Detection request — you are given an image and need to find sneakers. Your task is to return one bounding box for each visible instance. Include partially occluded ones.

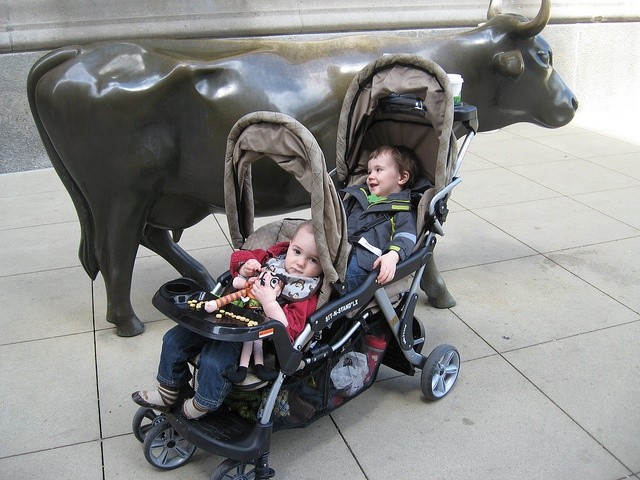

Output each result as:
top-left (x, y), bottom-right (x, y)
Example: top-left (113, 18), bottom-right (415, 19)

top-left (182, 397), bottom-right (208, 420)
top-left (132, 389), bottom-right (174, 414)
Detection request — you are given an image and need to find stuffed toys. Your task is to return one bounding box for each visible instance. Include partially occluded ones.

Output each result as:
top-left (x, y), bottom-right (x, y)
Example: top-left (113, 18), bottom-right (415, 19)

top-left (204, 263), bottom-right (286, 383)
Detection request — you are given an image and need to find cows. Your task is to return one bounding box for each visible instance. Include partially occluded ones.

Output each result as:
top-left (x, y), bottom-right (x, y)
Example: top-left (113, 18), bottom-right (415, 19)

top-left (25, 0), bottom-right (579, 336)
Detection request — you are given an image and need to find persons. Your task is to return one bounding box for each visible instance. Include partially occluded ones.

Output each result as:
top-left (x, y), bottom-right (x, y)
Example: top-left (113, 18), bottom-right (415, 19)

top-left (343, 144), bottom-right (421, 292)
top-left (131, 220), bottom-right (324, 420)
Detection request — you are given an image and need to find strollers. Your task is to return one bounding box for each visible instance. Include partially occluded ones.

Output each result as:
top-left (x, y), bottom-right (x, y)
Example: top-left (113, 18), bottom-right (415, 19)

top-left (132, 53), bottom-right (480, 479)
